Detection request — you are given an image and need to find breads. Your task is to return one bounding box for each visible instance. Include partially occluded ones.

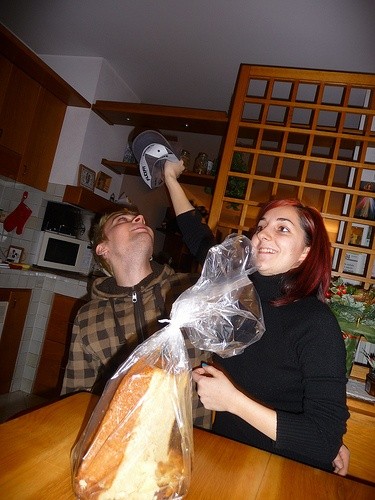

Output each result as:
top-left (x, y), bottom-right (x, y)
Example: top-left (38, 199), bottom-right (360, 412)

top-left (73, 352), bottom-right (186, 500)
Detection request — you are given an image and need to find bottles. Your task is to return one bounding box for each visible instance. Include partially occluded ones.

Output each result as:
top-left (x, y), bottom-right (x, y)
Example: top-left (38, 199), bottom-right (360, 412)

top-left (365, 368), bottom-right (375, 396)
top-left (205, 159), bottom-right (220, 175)
top-left (193, 152), bottom-right (207, 175)
top-left (180, 151), bottom-right (191, 175)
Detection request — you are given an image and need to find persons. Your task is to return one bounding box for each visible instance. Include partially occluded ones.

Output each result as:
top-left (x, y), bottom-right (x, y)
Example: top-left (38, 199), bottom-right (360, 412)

top-left (61, 209), bottom-right (350, 476)
top-left (164, 160), bottom-right (350, 472)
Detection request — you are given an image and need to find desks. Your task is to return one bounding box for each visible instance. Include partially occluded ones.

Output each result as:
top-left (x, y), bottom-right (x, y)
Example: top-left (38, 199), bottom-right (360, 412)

top-left (0, 391), bottom-right (375, 500)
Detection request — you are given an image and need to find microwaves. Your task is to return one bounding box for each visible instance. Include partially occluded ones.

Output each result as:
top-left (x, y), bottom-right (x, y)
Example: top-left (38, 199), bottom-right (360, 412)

top-left (36, 230), bottom-right (96, 276)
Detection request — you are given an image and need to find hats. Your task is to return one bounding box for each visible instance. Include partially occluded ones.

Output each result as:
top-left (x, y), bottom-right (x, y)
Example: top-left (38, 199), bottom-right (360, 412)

top-left (131, 129), bottom-right (179, 190)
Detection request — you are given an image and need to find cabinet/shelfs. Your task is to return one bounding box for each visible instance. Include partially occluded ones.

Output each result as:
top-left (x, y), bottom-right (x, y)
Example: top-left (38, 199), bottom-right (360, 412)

top-left (0, 25), bottom-right (92, 191)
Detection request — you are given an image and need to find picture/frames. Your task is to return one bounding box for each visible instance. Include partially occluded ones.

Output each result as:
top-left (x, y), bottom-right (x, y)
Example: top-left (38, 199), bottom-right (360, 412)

top-left (78, 164), bottom-right (96, 192)
top-left (4, 245), bottom-right (25, 264)
